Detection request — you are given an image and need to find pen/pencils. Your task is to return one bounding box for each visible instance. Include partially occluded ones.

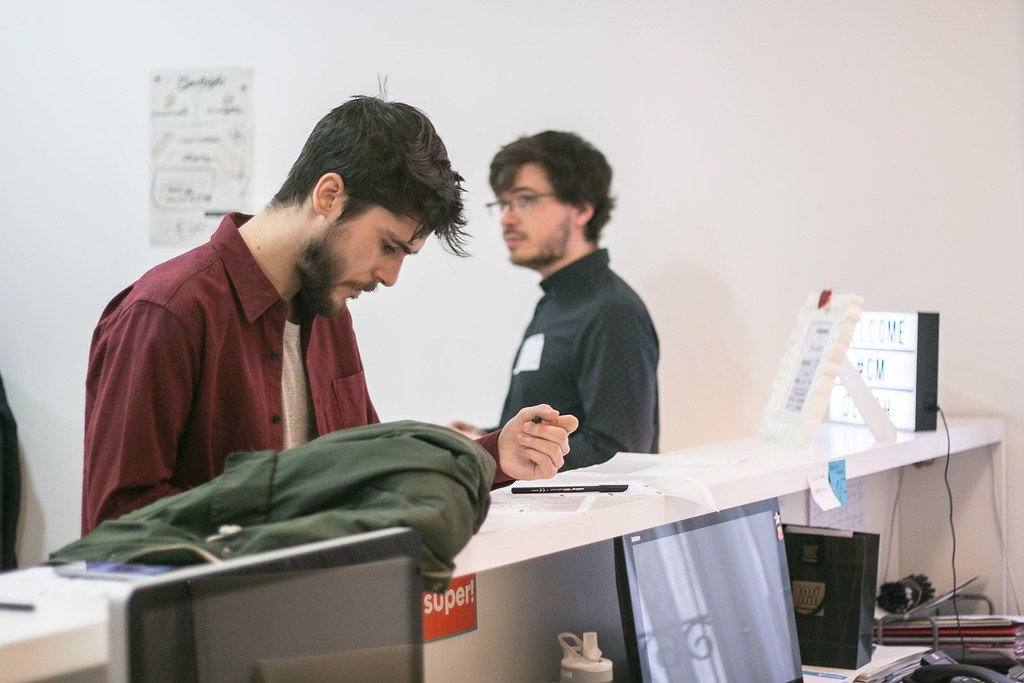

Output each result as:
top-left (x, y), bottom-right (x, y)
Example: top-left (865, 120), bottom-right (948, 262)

top-left (531, 416), bottom-right (542, 424)
top-left (0, 603), bottom-right (33, 612)
top-left (511, 485), bottom-right (629, 494)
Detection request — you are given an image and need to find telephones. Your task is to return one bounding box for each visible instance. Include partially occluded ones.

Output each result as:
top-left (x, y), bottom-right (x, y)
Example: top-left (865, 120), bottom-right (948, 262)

top-left (902, 650), bottom-right (1024, 683)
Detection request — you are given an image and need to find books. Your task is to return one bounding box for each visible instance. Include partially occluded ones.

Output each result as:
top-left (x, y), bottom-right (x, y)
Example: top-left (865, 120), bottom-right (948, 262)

top-left (802, 614), bottom-right (1024, 683)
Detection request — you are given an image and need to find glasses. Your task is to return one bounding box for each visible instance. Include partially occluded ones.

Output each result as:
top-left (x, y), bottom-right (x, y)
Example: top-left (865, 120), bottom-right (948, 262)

top-left (486, 192), bottom-right (557, 216)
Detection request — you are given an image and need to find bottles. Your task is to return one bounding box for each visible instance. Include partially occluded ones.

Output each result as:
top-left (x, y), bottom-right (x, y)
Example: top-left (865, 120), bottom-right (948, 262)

top-left (557, 629), bottom-right (613, 683)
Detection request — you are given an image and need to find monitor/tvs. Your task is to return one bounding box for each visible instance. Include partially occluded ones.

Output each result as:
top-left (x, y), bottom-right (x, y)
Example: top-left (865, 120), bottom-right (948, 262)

top-left (614, 496), bottom-right (806, 683)
top-left (105, 525), bottom-right (430, 683)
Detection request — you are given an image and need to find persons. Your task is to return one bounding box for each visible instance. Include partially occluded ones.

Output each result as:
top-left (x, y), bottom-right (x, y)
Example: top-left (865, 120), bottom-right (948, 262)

top-left (446, 130), bottom-right (660, 474)
top-left (81, 74), bottom-right (578, 537)
top-left (0, 375), bottom-right (20, 574)
